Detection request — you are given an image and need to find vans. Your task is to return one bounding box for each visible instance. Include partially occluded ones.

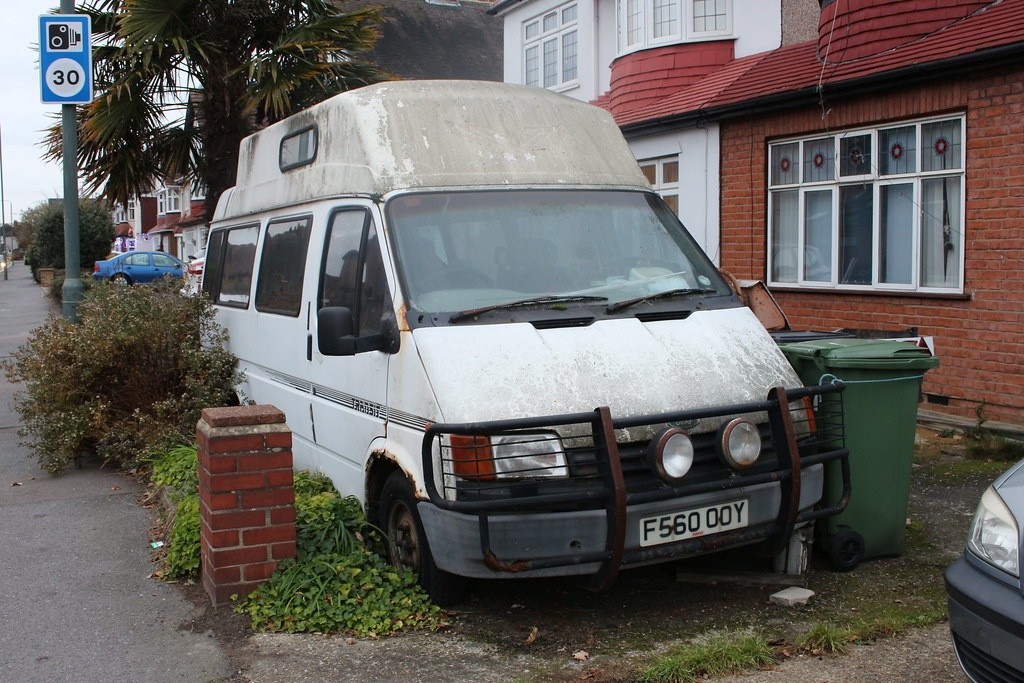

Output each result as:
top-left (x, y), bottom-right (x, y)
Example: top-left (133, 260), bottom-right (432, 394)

top-left (197, 80), bottom-right (824, 604)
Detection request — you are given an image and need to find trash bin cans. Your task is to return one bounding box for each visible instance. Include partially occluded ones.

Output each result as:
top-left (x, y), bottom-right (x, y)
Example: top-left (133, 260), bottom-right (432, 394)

top-left (774, 338), bottom-right (939, 573)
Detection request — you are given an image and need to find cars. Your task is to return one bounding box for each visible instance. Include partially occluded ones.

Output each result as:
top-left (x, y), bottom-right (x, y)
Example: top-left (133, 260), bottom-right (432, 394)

top-left (93, 251), bottom-right (190, 287)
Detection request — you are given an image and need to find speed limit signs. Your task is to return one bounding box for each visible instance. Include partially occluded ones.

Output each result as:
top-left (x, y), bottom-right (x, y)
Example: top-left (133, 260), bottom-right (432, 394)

top-left (39, 14), bottom-right (94, 105)
